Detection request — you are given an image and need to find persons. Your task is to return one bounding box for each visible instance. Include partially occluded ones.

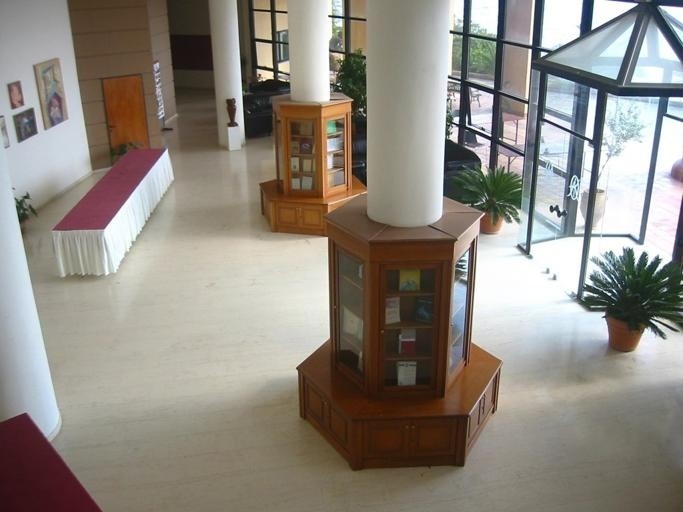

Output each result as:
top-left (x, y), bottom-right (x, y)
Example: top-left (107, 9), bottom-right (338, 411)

top-left (9, 83), bottom-right (22, 109)
top-left (48, 95), bottom-right (63, 124)
top-left (14, 114), bottom-right (33, 139)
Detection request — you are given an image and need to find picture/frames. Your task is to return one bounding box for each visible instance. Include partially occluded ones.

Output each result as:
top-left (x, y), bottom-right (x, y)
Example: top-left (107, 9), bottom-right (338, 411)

top-left (5, 57), bottom-right (70, 143)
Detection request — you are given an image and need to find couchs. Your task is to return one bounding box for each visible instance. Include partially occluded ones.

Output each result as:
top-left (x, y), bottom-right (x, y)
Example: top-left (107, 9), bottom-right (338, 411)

top-left (242, 78), bottom-right (291, 136)
top-left (353, 136), bottom-right (481, 206)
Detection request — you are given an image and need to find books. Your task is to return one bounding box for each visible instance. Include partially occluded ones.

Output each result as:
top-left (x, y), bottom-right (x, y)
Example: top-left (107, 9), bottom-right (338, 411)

top-left (286, 120), bottom-right (340, 192)
top-left (398, 269), bottom-right (421, 291)
top-left (342, 307), bottom-right (363, 346)
top-left (355, 351), bottom-right (364, 371)
top-left (396, 360), bottom-right (417, 386)
top-left (384, 297), bottom-right (400, 324)
top-left (398, 329), bottom-right (415, 358)
top-left (413, 297), bottom-right (433, 325)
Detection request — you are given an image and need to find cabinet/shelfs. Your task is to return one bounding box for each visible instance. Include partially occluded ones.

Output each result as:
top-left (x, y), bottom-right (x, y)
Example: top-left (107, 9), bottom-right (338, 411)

top-left (324, 224), bottom-right (476, 401)
top-left (267, 95), bottom-right (351, 199)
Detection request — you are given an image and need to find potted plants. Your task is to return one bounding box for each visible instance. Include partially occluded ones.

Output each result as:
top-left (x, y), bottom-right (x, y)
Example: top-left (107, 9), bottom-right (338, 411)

top-left (579, 97), bottom-right (646, 232)
top-left (450, 162), bottom-right (524, 234)
top-left (570, 245), bottom-right (683, 353)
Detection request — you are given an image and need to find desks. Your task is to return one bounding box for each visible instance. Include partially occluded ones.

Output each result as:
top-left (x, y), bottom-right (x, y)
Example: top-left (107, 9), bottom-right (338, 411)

top-left (51, 146), bottom-right (171, 280)
